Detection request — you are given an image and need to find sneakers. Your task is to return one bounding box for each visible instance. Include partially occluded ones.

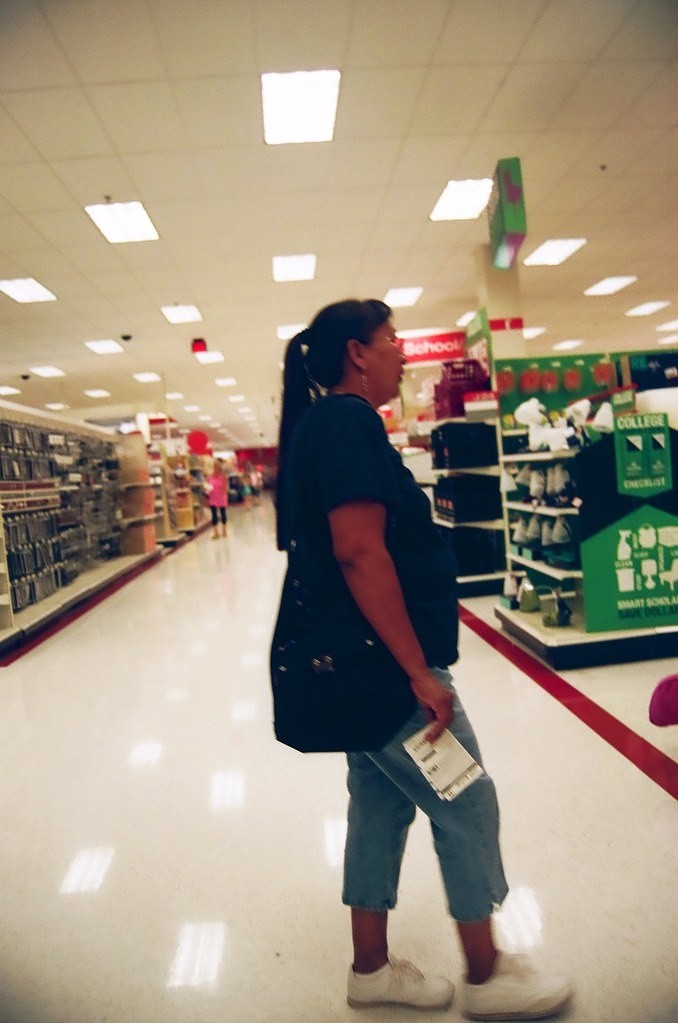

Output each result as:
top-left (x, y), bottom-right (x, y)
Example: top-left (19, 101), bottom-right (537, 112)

top-left (346, 953), bottom-right (455, 1010)
top-left (460, 949), bottom-right (572, 1021)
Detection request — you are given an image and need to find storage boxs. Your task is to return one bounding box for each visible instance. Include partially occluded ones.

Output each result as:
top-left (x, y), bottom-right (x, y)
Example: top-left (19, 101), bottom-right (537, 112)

top-left (116, 466), bottom-right (157, 554)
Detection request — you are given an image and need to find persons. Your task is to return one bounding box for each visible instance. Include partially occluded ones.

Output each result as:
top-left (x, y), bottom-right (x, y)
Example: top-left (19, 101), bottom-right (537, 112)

top-left (213, 298), bottom-right (576, 1022)
top-left (202, 457), bottom-right (263, 541)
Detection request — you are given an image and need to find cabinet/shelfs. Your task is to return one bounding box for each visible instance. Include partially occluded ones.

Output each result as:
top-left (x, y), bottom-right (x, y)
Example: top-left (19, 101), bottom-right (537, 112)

top-left (148, 443), bottom-right (224, 544)
top-left (375, 304), bottom-right (678, 671)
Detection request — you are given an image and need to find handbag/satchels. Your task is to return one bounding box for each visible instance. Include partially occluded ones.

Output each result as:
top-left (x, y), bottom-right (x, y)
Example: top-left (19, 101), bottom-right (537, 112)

top-left (271, 529), bottom-right (418, 753)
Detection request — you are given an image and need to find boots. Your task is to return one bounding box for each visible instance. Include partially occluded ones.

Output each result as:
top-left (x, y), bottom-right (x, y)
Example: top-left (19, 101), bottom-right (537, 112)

top-left (221, 524), bottom-right (227, 537)
top-left (211, 525), bottom-right (219, 540)
